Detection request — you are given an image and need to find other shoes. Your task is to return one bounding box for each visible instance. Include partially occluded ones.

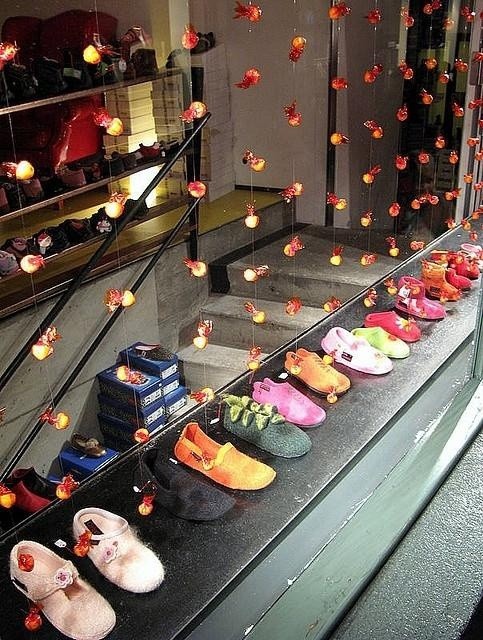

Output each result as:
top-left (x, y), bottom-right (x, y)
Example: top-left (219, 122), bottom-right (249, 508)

top-left (222, 392), bottom-right (312, 458)
top-left (365, 311), bottom-right (422, 343)
top-left (321, 328), bottom-right (394, 375)
top-left (284, 348), bottom-right (350, 395)
top-left (353, 325), bottom-right (411, 360)
top-left (252, 378), bottom-right (326, 428)
top-left (173, 420), bottom-right (278, 491)
top-left (432, 244), bottom-right (482, 290)
top-left (134, 447), bottom-right (236, 520)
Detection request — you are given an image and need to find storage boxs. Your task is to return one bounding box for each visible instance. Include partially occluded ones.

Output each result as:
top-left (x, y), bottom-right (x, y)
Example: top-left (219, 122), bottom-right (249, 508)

top-left (48, 338), bottom-right (188, 487)
top-left (103, 39), bottom-right (239, 203)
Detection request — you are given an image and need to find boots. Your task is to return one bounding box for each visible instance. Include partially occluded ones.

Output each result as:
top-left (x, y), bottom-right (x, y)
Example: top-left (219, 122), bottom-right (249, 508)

top-left (420, 261), bottom-right (461, 302)
top-left (2, 467), bottom-right (58, 512)
top-left (397, 276), bottom-right (448, 320)
top-left (0, 49), bottom-right (158, 105)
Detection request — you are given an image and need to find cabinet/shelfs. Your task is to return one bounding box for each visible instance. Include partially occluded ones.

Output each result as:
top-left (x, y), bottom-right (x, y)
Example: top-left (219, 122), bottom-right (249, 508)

top-left (0, 63), bottom-right (205, 320)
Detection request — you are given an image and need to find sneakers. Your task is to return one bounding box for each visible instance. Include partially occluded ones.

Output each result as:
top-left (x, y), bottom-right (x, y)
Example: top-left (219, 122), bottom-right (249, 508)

top-left (0, 140), bottom-right (179, 216)
top-left (134, 343), bottom-right (176, 361)
top-left (71, 432), bottom-right (106, 457)
top-left (0, 198), bottom-right (149, 276)
top-left (10, 506), bottom-right (165, 640)
